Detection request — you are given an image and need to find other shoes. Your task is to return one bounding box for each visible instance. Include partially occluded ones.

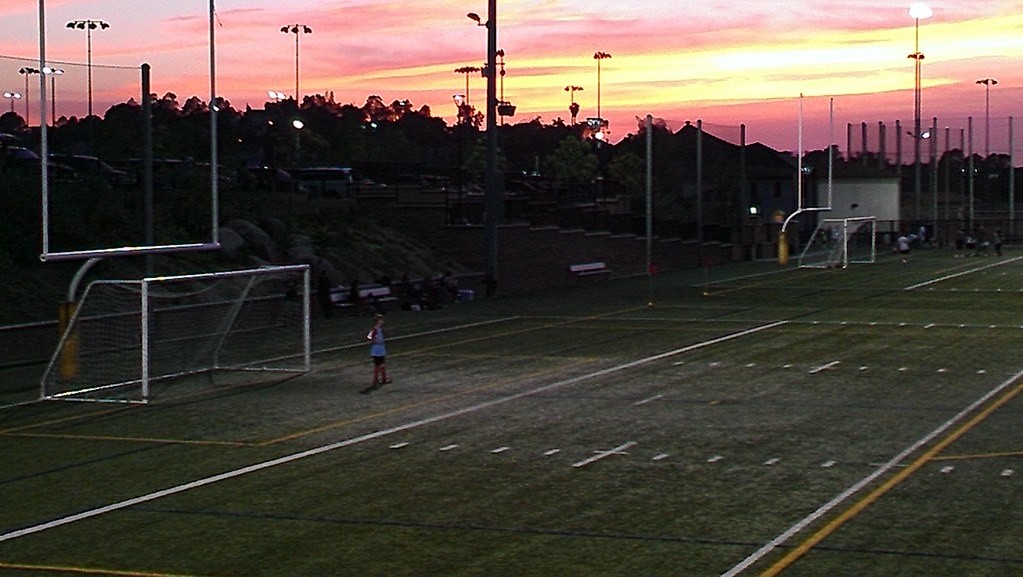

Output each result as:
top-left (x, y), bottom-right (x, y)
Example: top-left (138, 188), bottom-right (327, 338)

top-left (381, 378), bottom-right (392, 384)
top-left (374, 381), bottom-right (384, 387)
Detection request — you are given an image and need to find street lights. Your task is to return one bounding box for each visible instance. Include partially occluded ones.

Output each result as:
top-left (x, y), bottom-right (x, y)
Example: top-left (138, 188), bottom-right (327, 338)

top-left (564, 85), bottom-right (585, 125)
top-left (975, 78), bottom-right (998, 158)
top-left (18, 65), bottom-right (64, 127)
top-left (594, 50), bottom-right (614, 118)
top-left (907, 3), bottom-right (934, 222)
top-left (64, 16), bottom-right (110, 115)
top-left (279, 22), bottom-right (313, 165)
top-left (467, 12), bottom-right (501, 302)
top-left (3, 92), bottom-right (22, 113)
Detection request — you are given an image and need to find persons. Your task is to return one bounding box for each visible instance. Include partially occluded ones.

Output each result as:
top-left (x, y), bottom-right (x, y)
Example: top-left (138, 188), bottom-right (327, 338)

top-left (400, 274), bottom-right (426, 310)
top-left (319, 270), bottom-right (339, 319)
top-left (897, 233), bottom-right (910, 263)
top-left (954, 228), bottom-right (965, 258)
top-left (993, 231), bottom-right (1002, 256)
top-left (883, 231), bottom-right (892, 249)
top-left (365, 314), bottom-right (392, 386)
top-left (349, 280), bottom-right (388, 317)
top-left (965, 230), bottom-right (978, 256)
top-left (440, 271), bottom-right (459, 303)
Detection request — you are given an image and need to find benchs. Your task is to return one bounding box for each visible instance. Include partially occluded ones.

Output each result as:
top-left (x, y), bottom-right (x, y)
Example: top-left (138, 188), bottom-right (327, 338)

top-left (331, 287), bottom-right (400, 317)
top-left (569, 262), bottom-right (613, 281)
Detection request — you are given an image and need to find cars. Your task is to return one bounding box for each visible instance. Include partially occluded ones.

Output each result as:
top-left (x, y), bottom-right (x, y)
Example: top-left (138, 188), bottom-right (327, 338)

top-left (1, 130), bottom-right (605, 213)
